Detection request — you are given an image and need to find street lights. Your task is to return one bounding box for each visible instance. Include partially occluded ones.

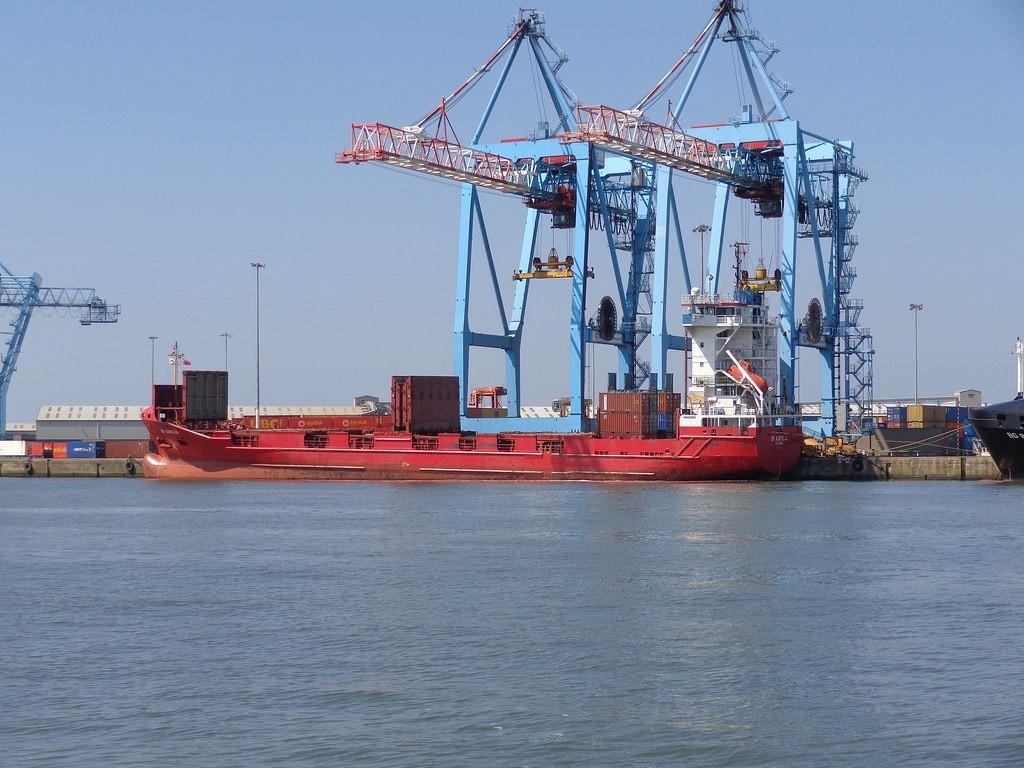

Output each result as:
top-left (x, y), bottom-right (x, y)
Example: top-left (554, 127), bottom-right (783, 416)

top-left (251, 260), bottom-right (266, 429)
top-left (150, 336), bottom-right (159, 385)
top-left (694, 225), bottom-right (710, 296)
top-left (909, 303), bottom-right (923, 405)
top-left (221, 333), bottom-right (233, 371)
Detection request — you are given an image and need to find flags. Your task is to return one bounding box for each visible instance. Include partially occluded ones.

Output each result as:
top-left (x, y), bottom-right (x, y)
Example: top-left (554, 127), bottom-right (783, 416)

top-left (183, 358), bottom-right (191, 365)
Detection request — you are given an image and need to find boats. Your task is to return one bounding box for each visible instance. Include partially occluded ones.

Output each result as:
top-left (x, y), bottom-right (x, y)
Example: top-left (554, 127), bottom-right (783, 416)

top-left (140, 341), bottom-right (804, 484)
top-left (967, 338), bottom-right (1024, 482)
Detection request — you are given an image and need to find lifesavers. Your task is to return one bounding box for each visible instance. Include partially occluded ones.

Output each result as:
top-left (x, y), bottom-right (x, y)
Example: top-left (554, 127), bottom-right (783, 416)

top-left (851, 460), bottom-right (864, 472)
